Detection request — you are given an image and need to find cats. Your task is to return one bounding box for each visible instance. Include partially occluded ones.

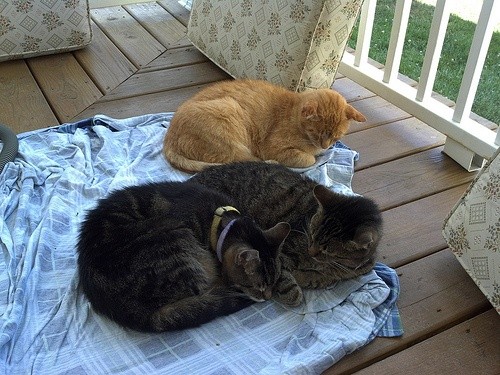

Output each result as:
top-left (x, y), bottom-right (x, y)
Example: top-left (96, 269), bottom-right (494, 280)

top-left (161, 80), bottom-right (367, 172)
top-left (74, 159), bottom-right (384, 334)
